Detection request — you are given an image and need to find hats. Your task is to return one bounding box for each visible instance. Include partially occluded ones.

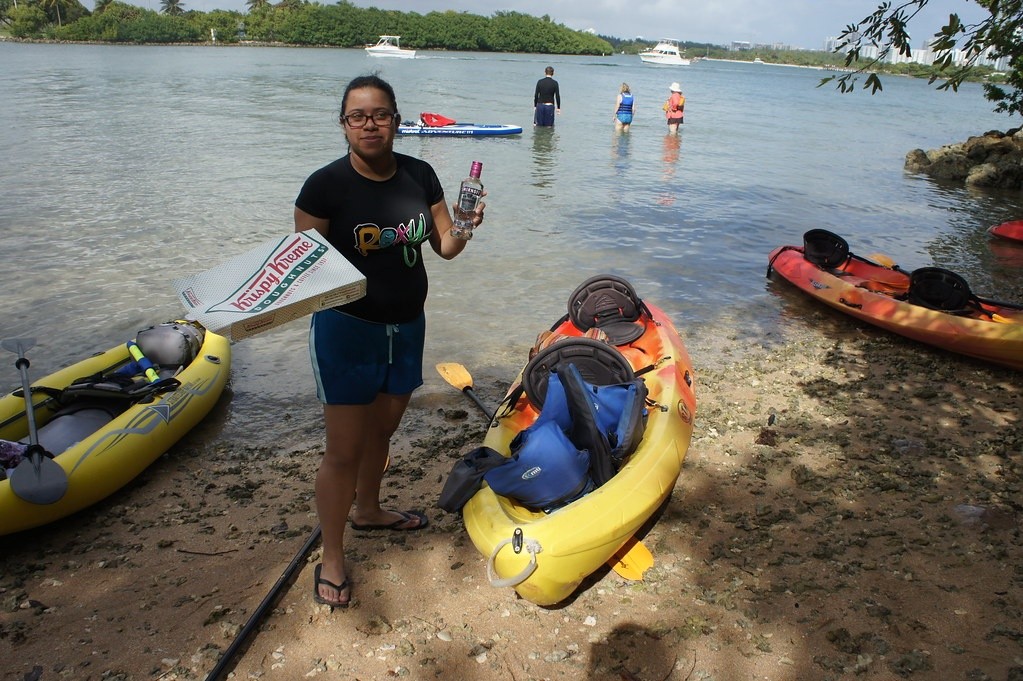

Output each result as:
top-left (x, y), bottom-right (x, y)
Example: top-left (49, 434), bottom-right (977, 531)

top-left (669, 83), bottom-right (682, 93)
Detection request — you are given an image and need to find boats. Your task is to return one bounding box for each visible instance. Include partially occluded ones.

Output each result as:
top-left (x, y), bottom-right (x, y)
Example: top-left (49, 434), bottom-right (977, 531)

top-left (986, 219), bottom-right (1023, 244)
top-left (395, 111), bottom-right (524, 140)
top-left (765, 227), bottom-right (1023, 374)
top-left (459, 272), bottom-right (698, 610)
top-left (0, 321), bottom-right (230, 539)
top-left (364, 33), bottom-right (417, 59)
top-left (639, 37), bottom-right (691, 67)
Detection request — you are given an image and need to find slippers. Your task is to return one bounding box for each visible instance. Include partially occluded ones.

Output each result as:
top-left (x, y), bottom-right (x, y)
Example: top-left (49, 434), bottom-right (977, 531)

top-left (352, 509), bottom-right (428, 530)
top-left (313, 563), bottom-right (351, 606)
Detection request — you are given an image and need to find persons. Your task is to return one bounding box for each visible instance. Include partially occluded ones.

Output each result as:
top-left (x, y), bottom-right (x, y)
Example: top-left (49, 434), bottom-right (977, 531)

top-left (665, 82), bottom-right (686, 134)
top-left (612, 81), bottom-right (636, 130)
top-left (534, 65), bottom-right (561, 125)
top-left (295, 74), bottom-right (490, 605)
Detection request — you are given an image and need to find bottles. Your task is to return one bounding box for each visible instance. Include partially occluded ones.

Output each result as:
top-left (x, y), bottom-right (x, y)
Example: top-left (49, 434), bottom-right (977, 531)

top-left (450, 160), bottom-right (484, 240)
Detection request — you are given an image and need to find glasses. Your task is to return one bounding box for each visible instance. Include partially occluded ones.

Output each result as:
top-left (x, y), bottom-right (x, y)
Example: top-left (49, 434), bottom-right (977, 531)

top-left (341, 112), bottom-right (394, 128)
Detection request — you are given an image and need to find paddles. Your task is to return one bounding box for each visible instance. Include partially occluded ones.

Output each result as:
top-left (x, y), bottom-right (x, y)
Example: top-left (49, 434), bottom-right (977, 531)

top-left (867, 252), bottom-right (1014, 326)
top-left (436, 357), bottom-right (656, 585)
top-left (201, 451), bottom-right (395, 681)
